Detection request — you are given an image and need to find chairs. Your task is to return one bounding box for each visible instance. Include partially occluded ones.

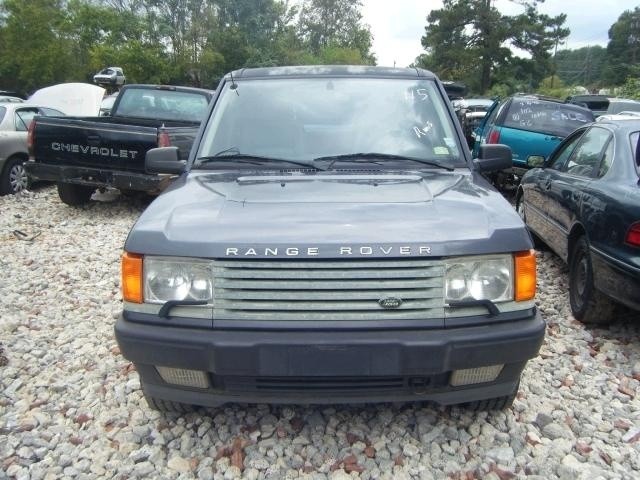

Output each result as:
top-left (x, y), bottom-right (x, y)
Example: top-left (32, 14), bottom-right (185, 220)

top-left (241, 105), bottom-right (308, 158)
